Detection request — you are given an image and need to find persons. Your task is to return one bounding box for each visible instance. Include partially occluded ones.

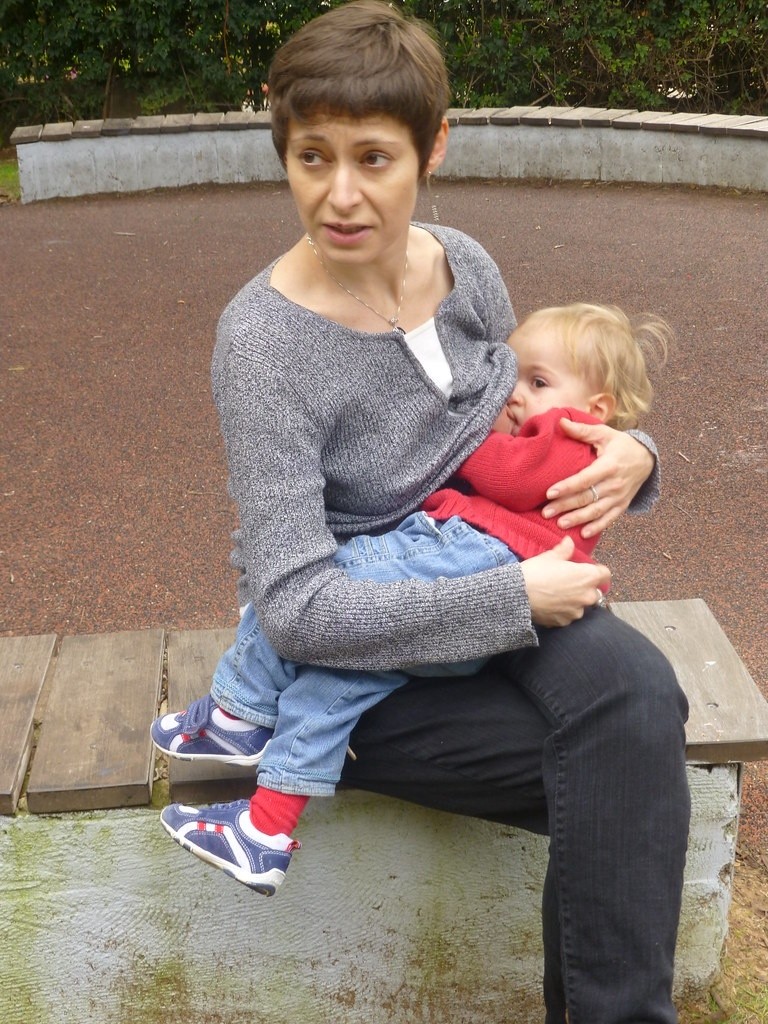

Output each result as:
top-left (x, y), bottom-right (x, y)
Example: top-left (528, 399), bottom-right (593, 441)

top-left (149, 298), bottom-right (671, 894)
top-left (207, 0), bottom-right (691, 1024)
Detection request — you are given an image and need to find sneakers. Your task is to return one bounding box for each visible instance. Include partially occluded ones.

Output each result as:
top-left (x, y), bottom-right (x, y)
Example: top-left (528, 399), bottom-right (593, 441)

top-left (150, 694), bottom-right (272, 764)
top-left (160, 799), bottom-right (301, 899)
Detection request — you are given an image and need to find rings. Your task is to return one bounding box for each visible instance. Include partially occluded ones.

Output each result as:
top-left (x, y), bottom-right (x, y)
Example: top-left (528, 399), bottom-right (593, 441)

top-left (590, 486), bottom-right (600, 501)
top-left (597, 588), bottom-right (605, 608)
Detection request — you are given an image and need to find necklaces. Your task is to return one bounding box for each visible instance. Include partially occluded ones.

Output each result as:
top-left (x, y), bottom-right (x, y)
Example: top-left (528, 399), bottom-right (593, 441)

top-left (307, 229), bottom-right (414, 334)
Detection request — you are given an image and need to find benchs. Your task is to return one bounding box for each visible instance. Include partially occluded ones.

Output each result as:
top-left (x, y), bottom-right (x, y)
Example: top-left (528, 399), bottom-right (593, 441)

top-left (1, 598), bottom-right (767, 1024)
top-left (11, 107), bottom-right (768, 203)
